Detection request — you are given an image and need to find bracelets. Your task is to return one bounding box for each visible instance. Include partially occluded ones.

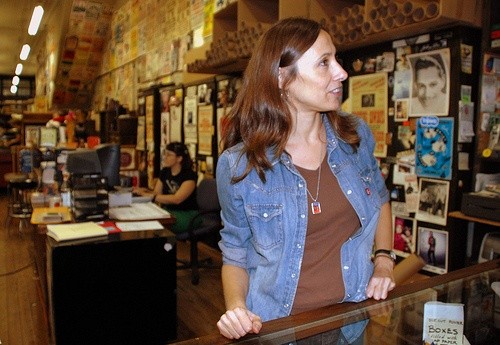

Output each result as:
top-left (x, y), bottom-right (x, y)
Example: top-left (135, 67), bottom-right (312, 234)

top-left (375, 249), bottom-right (396, 260)
top-left (152, 194), bottom-right (157, 202)
top-left (371, 255), bottom-right (393, 263)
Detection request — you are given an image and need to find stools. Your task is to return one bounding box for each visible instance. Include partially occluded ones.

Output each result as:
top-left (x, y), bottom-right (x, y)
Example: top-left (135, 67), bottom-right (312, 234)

top-left (5, 171), bottom-right (35, 219)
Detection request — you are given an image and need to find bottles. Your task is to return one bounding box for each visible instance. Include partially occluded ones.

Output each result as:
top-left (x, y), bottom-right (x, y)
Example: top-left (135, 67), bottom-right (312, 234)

top-left (61, 175), bottom-right (72, 212)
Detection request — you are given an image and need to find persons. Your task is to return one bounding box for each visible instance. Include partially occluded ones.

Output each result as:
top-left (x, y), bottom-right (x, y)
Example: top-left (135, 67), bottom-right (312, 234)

top-left (133, 141), bottom-right (203, 233)
top-left (427, 231), bottom-right (437, 266)
top-left (394, 218), bottom-right (412, 253)
top-left (215, 16), bottom-right (396, 345)
top-left (395, 49), bottom-right (448, 115)
top-left (419, 185), bottom-right (446, 217)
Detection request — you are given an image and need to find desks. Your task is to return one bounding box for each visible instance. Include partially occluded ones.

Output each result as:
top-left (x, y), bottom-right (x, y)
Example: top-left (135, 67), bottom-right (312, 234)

top-left (32, 219), bottom-right (177, 344)
top-left (448, 210), bottom-right (500, 257)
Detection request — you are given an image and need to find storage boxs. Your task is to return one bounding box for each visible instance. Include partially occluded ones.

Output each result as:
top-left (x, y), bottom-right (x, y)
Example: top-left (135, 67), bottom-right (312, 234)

top-left (464, 190), bottom-right (500, 222)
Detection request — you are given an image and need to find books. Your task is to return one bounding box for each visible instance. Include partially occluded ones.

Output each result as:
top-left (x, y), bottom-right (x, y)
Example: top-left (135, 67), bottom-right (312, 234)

top-left (45, 220), bottom-right (109, 248)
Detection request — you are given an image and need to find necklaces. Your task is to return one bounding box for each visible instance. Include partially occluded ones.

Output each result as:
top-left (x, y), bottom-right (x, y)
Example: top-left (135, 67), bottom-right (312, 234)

top-left (287, 124), bottom-right (325, 215)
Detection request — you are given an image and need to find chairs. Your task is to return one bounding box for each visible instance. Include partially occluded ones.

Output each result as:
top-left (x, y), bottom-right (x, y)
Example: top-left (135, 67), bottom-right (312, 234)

top-left (176, 178), bottom-right (223, 286)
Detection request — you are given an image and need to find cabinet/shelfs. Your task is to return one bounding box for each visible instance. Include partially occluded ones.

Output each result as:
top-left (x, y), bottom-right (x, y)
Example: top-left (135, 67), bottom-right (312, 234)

top-left (186, 0), bottom-right (483, 75)
top-left (98, 109), bottom-right (137, 145)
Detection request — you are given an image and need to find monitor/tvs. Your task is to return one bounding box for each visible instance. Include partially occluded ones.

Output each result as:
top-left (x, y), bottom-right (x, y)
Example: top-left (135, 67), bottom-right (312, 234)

top-left (66, 143), bottom-right (121, 192)
